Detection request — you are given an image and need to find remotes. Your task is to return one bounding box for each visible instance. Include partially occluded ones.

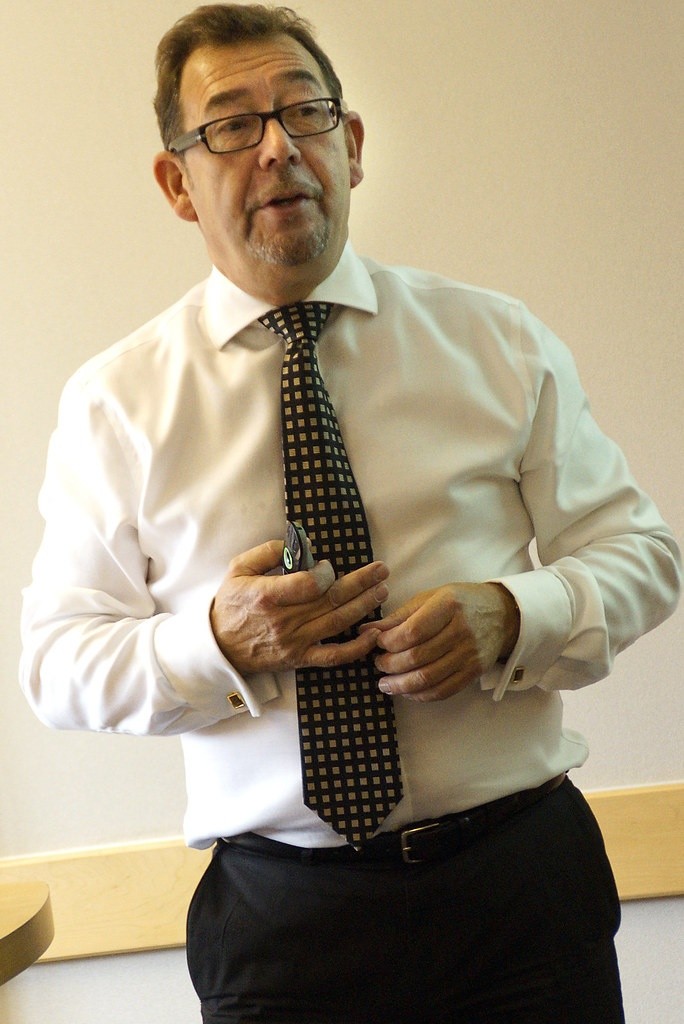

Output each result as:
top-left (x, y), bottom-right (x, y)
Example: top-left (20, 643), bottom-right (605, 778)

top-left (281, 521), bottom-right (315, 577)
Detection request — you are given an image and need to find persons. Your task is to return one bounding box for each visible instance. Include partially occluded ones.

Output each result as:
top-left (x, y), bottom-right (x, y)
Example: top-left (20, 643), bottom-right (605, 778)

top-left (20, 5), bottom-right (682, 1024)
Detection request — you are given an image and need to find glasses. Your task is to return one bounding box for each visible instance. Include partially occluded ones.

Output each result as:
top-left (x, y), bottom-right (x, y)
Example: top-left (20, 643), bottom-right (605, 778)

top-left (164, 96), bottom-right (343, 154)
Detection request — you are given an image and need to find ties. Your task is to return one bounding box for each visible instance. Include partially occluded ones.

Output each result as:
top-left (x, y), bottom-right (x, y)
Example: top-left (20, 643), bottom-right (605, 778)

top-left (258, 299), bottom-right (407, 853)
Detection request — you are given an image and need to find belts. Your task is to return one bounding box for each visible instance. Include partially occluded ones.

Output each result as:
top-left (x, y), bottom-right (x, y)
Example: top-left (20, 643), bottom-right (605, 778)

top-left (215, 772), bottom-right (569, 865)
